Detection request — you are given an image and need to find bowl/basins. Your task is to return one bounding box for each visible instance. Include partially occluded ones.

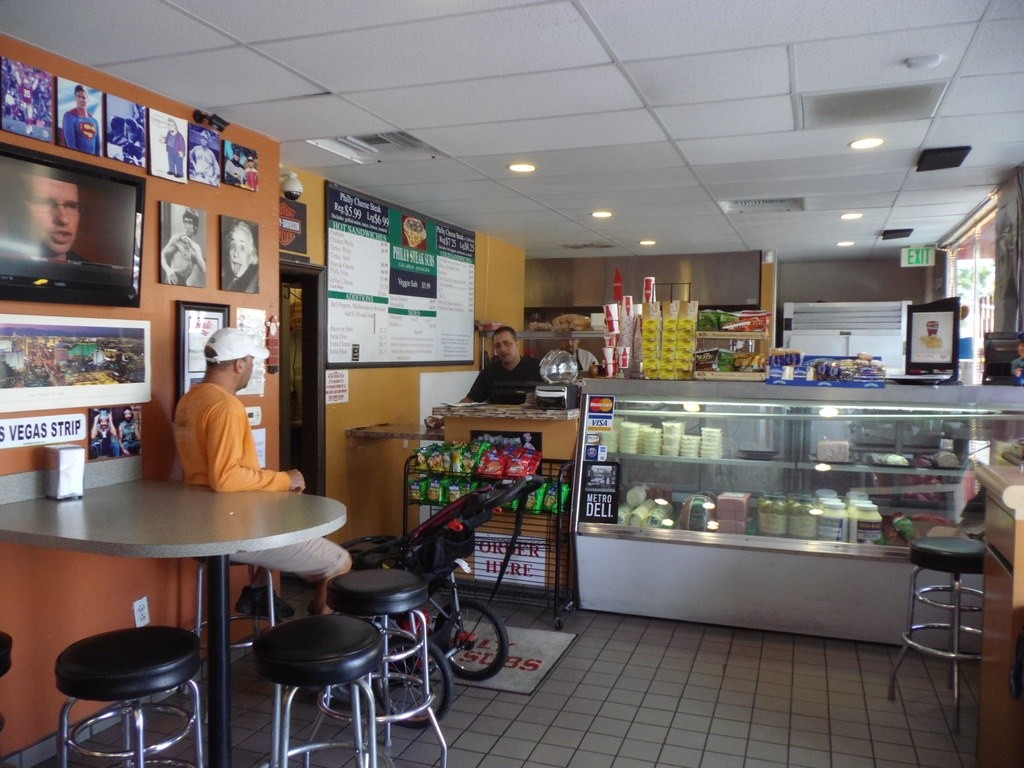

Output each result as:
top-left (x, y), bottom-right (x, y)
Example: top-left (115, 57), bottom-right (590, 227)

top-left (602, 417), bottom-right (723, 460)
top-left (738, 450), bottom-right (780, 460)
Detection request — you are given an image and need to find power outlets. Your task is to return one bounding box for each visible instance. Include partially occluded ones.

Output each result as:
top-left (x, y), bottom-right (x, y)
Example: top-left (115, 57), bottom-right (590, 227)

top-left (133, 596), bottom-right (151, 627)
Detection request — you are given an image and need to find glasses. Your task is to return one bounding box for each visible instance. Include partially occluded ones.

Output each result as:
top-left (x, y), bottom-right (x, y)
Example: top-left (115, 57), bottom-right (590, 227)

top-left (23, 197), bottom-right (84, 216)
top-left (247, 159), bottom-right (254, 162)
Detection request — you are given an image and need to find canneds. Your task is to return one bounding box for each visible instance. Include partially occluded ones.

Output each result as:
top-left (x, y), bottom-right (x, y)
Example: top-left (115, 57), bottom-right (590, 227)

top-left (757, 490), bottom-right (881, 544)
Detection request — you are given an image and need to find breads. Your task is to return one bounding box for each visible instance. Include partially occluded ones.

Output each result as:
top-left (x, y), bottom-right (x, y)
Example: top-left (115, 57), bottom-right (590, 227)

top-left (769, 346), bottom-right (884, 380)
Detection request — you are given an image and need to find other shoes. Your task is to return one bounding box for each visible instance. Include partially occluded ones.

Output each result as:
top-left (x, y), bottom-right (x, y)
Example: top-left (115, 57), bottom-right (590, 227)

top-left (234, 585), bottom-right (295, 619)
top-left (304, 683), bottom-right (364, 704)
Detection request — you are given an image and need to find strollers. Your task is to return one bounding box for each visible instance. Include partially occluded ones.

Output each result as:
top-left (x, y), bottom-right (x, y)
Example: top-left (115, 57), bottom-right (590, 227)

top-left (337, 473), bottom-right (544, 730)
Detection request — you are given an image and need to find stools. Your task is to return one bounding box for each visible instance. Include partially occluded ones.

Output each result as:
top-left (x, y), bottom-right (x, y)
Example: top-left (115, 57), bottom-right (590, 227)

top-left (306, 569), bottom-right (448, 768)
top-left (55, 625), bottom-right (206, 768)
top-left (249, 614), bottom-right (383, 768)
top-left (887, 536), bottom-right (987, 739)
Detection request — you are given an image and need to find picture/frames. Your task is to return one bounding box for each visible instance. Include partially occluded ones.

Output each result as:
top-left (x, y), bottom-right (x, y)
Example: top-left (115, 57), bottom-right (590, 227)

top-left (173, 298), bottom-right (231, 427)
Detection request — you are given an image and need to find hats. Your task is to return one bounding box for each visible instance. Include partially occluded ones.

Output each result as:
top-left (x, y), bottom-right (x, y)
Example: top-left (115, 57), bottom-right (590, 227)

top-left (204, 326), bottom-right (270, 363)
top-left (201, 130), bottom-right (210, 140)
top-left (234, 149), bottom-right (240, 157)
top-left (98, 410), bottom-right (110, 419)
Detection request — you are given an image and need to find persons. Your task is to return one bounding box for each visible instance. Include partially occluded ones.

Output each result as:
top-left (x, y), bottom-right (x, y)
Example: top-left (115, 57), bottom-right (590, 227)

top-left (222, 220), bottom-right (258, 293)
top-left (0, 170), bottom-right (104, 268)
top-left (90, 409), bottom-right (141, 459)
top-left (161, 209), bottom-right (206, 286)
top-left (106, 117), bottom-right (143, 166)
top-left (13, 66), bottom-right (39, 135)
top-left (174, 327), bottom-right (352, 622)
top-left (549, 332), bottom-right (599, 379)
top-left (63, 85), bottom-right (100, 157)
top-left (424, 326), bottom-right (551, 430)
top-left (189, 130), bottom-right (220, 182)
top-left (225, 148), bottom-right (258, 188)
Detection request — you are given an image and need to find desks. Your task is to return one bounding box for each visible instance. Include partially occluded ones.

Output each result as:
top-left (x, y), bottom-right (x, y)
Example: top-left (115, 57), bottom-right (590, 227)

top-left (1, 480), bottom-right (347, 768)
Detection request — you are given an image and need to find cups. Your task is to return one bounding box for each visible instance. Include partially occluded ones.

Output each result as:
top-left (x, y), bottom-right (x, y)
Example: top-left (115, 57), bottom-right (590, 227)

top-left (601, 276), bottom-right (657, 379)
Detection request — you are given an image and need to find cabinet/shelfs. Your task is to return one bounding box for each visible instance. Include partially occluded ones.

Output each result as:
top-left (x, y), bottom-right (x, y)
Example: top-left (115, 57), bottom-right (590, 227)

top-left (480, 326), bottom-right (772, 380)
top-left (974, 461), bottom-right (1023, 768)
top-left (570, 380), bottom-right (1024, 647)
